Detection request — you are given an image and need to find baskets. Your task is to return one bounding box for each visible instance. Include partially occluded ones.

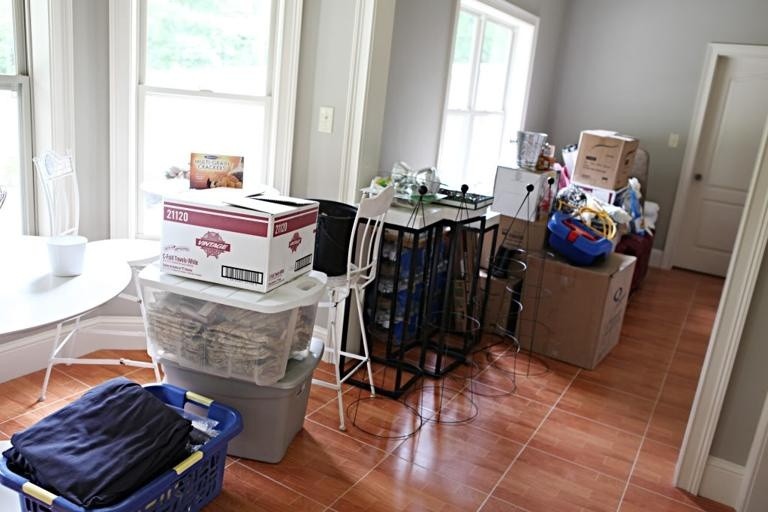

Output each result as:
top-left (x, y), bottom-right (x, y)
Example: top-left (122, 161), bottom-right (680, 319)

top-left (0, 382), bottom-right (244, 511)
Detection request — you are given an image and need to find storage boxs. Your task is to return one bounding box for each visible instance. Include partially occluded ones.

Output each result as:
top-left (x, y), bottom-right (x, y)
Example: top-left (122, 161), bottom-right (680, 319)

top-left (482, 130), bottom-right (638, 370)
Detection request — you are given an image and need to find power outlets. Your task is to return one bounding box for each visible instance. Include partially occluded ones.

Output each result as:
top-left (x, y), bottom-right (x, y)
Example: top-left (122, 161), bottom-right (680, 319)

top-left (317, 107), bottom-right (334, 134)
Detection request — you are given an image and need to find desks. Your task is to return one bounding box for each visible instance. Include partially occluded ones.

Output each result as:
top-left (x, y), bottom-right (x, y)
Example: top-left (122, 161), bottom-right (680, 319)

top-left (340, 205), bottom-right (501, 401)
top-left (0, 234), bottom-right (132, 336)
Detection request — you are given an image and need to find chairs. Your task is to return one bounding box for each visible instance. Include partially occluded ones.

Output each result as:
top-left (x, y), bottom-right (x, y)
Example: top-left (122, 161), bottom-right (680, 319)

top-left (32, 145), bottom-right (166, 402)
top-left (311, 185), bottom-right (394, 431)
